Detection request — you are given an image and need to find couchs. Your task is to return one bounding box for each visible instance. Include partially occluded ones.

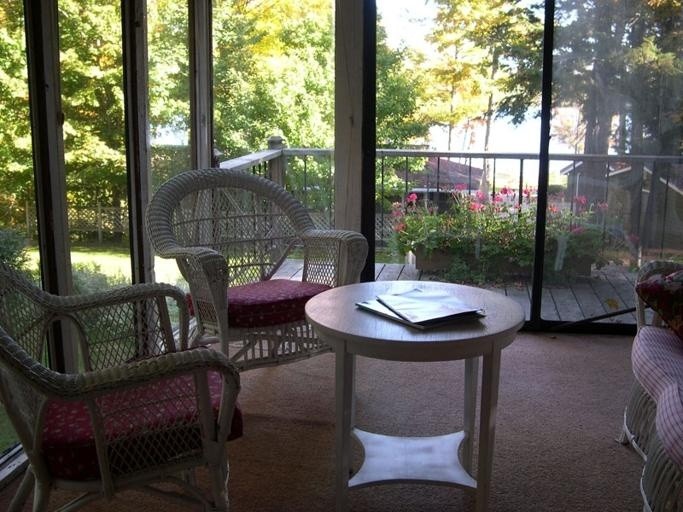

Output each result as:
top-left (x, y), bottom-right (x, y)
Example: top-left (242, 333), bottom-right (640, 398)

top-left (615, 270), bottom-right (683, 512)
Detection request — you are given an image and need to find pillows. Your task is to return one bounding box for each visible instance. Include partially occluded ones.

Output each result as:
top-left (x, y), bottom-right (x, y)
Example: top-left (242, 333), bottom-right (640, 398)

top-left (636, 271), bottom-right (682, 336)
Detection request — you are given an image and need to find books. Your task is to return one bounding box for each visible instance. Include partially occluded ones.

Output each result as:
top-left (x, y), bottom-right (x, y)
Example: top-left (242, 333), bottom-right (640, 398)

top-left (354, 288), bottom-right (486, 331)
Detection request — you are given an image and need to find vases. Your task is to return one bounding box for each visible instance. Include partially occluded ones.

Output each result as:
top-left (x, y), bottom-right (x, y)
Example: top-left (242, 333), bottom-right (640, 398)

top-left (408, 241), bottom-right (593, 279)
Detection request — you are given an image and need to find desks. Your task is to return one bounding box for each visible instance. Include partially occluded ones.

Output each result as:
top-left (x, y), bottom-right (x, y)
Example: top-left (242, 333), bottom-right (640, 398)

top-left (306, 281), bottom-right (525, 511)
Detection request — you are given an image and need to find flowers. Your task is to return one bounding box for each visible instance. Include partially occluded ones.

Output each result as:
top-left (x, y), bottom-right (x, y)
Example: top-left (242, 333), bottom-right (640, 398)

top-left (389, 184), bottom-right (639, 284)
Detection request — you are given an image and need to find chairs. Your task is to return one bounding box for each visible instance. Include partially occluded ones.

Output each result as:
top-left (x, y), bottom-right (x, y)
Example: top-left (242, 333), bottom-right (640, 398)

top-left (1, 259), bottom-right (245, 512)
top-left (146, 169), bottom-right (369, 429)
